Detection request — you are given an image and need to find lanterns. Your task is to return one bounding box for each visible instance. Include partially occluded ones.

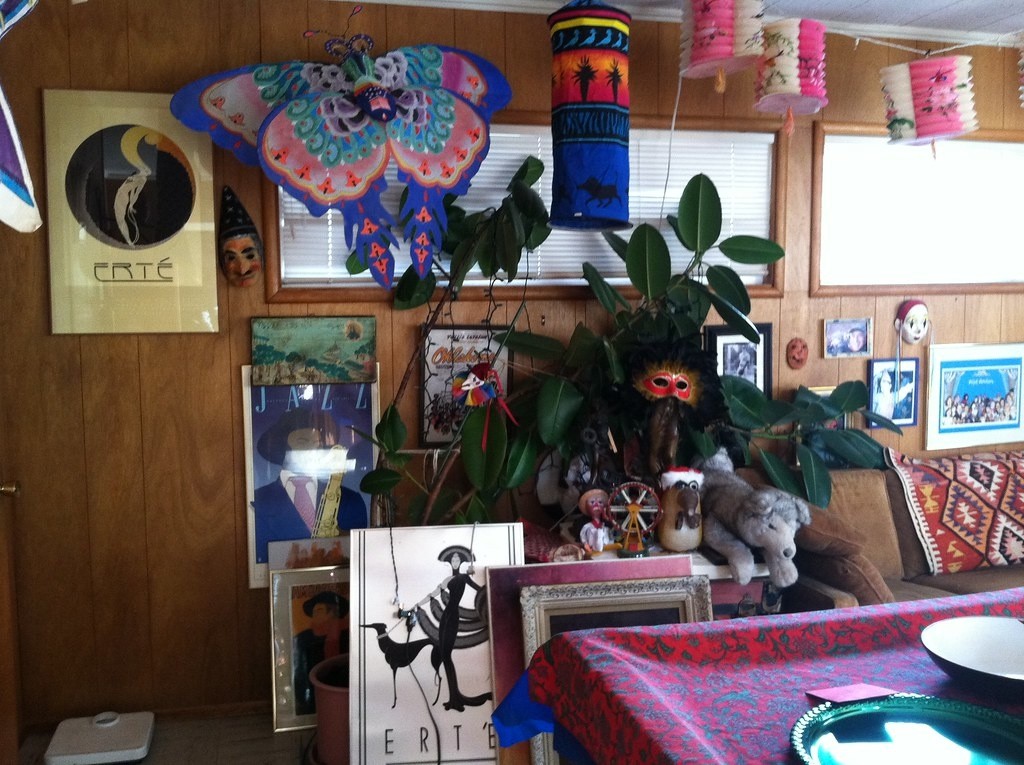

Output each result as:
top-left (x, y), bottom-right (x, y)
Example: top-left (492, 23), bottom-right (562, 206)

top-left (680, 0), bottom-right (763, 77)
top-left (879, 55), bottom-right (980, 147)
top-left (546, 0), bottom-right (632, 234)
top-left (755, 18), bottom-right (828, 117)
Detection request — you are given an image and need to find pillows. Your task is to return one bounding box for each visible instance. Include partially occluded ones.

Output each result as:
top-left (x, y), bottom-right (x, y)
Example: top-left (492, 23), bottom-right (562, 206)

top-left (751, 483), bottom-right (866, 556)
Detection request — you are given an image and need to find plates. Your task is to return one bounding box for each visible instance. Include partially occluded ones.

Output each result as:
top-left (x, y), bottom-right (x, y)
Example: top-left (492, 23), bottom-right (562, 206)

top-left (790, 694), bottom-right (1024, 765)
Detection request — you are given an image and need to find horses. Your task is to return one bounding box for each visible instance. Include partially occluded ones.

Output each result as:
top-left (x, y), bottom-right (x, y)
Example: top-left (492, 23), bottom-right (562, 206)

top-left (580, 176), bottom-right (623, 208)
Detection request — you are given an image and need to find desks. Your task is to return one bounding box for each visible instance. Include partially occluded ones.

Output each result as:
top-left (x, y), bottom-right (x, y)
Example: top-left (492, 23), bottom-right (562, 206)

top-left (488, 586), bottom-right (1024, 765)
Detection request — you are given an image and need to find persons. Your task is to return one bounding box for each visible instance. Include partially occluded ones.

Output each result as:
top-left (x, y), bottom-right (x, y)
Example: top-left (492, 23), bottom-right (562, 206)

top-left (833, 325), bottom-right (868, 353)
top-left (577, 486), bottom-right (619, 555)
top-left (943, 386), bottom-right (1018, 427)
top-left (734, 344), bottom-right (751, 379)
top-left (409, 544), bottom-right (498, 710)
top-left (873, 368), bottom-right (913, 424)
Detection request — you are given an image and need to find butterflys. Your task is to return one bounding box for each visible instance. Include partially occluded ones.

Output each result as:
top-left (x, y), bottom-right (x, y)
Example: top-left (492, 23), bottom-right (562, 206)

top-left (169, 6), bottom-right (512, 289)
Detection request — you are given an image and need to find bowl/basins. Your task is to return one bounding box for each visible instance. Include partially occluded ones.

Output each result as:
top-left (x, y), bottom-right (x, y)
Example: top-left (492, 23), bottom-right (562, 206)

top-left (920, 615), bottom-right (1024, 698)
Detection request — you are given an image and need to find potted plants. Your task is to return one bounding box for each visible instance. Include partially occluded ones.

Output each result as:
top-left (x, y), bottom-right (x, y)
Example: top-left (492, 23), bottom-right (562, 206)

top-left (311, 154), bottom-right (904, 765)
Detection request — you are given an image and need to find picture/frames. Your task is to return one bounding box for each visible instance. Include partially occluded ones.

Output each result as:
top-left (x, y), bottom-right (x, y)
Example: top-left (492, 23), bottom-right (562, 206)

top-left (242, 363), bottom-right (385, 589)
top-left (796, 386), bottom-right (848, 466)
top-left (924, 343), bottom-right (1024, 450)
top-left (822, 317), bottom-right (873, 360)
top-left (269, 565), bottom-right (365, 734)
top-left (520, 574), bottom-right (714, 765)
top-left (703, 323), bottom-right (773, 403)
top-left (418, 323), bottom-right (514, 448)
top-left (869, 358), bottom-right (920, 429)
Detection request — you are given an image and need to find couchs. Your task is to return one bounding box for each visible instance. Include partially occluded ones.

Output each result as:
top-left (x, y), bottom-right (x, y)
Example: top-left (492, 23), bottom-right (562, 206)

top-left (738, 450), bottom-right (1024, 614)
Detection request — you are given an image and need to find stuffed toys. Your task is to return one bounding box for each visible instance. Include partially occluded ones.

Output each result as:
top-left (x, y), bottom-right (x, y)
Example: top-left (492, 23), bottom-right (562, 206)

top-left (696, 447), bottom-right (807, 588)
